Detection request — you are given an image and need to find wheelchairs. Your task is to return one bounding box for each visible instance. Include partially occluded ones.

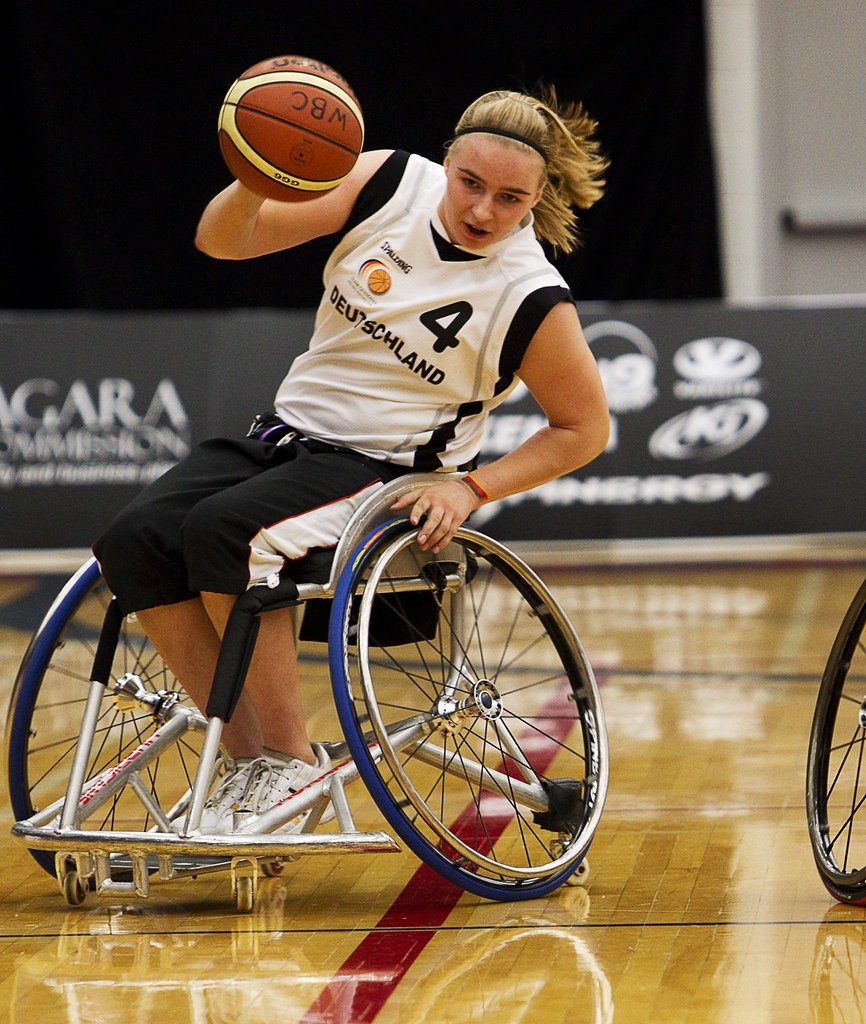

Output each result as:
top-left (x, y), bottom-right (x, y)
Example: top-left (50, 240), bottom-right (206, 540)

top-left (7, 466), bottom-right (609, 914)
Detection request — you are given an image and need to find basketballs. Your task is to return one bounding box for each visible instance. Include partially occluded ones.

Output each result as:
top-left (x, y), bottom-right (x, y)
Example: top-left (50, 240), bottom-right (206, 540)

top-left (213, 53), bottom-right (366, 204)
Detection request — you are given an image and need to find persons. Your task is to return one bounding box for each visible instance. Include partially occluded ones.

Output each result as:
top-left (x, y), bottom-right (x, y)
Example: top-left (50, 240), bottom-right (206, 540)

top-left (92, 87), bottom-right (611, 847)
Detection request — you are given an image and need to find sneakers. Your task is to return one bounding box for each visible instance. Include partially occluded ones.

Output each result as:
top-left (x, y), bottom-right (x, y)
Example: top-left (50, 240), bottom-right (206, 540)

top-left (169, 743), bottom-right (336, 835)
